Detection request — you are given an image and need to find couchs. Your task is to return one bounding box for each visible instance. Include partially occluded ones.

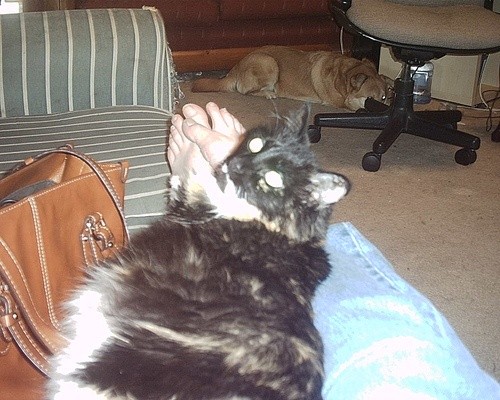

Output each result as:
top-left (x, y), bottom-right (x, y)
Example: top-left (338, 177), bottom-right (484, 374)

top-left (1, 7), bottom-right (261, 399)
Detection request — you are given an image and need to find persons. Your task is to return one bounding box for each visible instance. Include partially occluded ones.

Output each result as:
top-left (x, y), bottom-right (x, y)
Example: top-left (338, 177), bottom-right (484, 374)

top-left (167, 102), bottom-right (499, 400)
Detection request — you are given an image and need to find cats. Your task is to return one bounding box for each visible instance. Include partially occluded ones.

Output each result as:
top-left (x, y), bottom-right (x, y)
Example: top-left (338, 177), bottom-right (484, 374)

top-left (40, 102), bottom-right (351, 399)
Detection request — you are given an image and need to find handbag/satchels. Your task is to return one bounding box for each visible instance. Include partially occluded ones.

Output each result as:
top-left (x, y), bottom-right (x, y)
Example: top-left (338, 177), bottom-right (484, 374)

top-left (1, 140), bottom-right (130, 399)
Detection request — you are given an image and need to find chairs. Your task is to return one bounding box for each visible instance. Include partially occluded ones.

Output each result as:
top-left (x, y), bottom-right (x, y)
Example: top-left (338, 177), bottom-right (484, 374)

top-left (308, 0), bottom-right (500, 172)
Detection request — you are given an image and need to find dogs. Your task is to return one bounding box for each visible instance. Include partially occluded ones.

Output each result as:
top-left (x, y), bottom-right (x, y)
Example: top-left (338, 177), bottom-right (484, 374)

top-left (191, 45), bottom-right (397, 110)
